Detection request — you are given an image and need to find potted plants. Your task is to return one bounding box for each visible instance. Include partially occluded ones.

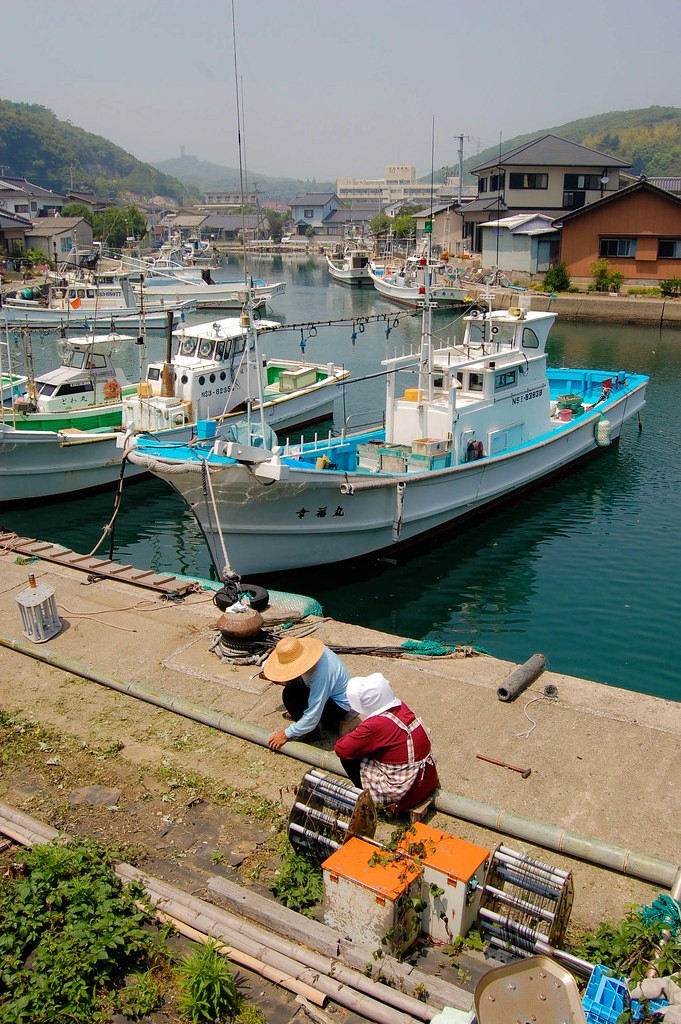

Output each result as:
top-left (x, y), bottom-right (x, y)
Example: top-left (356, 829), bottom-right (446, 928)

top-left (608, 282), bottom-right (665, 299)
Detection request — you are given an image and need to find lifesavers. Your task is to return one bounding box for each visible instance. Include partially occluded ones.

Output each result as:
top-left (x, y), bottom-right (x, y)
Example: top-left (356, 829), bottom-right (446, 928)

top-left (217, 584), bottom-right (269, 612)
top-left (104, 382), bottom-right (120, 399)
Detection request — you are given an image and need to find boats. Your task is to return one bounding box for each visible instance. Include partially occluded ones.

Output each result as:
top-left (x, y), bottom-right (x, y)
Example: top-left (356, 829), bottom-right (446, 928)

top-left (370, 230), bottom-right (480, 318)
top-left (116, 0), bottom-right (650, 581)
top-left (0, 194), bottom-right (350, 508)
top-left (326, 224), bottom-right (376, 285)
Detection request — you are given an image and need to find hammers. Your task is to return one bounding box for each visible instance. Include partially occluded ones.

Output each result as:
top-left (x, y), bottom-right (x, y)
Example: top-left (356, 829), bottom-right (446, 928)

top-left (476, 753), bottom-right (531, 778)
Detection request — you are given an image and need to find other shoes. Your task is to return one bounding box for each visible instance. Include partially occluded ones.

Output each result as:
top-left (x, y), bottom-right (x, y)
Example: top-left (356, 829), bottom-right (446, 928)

top-left (292, 726), bottom-right (321, 741)
top-left (282, 712), bottom-right (292, 719)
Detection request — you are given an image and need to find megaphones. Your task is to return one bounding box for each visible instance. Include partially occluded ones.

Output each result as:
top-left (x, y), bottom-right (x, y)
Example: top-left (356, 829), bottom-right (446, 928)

top-left (508, 307), bottom-right (528, 319)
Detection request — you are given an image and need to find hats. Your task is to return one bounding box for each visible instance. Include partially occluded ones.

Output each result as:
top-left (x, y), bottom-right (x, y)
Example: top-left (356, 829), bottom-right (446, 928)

top-left (346, 673), bottom-right (402, 721)
top-left (264, 637), bottom-right (324, 682)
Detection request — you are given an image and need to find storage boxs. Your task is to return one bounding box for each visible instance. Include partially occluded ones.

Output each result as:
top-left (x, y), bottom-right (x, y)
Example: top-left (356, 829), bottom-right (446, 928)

top-left (356, 436), bottom-right (451, 474)
top-left (581, 963), bottom-right (670, 1024)
top-left (555, 393), bottom-right (586, 418)
top-left (277, 364), bottom-right (319, 393)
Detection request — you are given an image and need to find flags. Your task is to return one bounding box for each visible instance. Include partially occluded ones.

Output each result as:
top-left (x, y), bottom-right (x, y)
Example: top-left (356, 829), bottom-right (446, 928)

top-left (70, 297), bottom-right (81, 310)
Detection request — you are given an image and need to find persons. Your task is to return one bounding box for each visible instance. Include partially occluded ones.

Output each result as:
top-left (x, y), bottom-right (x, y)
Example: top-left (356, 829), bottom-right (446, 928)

top-left (258, 636), bottom-right (352, 750)
top-left (335, 673), bottom-right (439, 819)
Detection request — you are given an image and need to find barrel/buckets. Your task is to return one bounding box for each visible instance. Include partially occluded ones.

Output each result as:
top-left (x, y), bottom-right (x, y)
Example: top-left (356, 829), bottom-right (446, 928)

top-left (561, 409), bottom-right (572, 421)
top-left (404, 388), bottom-right (423, 402)
